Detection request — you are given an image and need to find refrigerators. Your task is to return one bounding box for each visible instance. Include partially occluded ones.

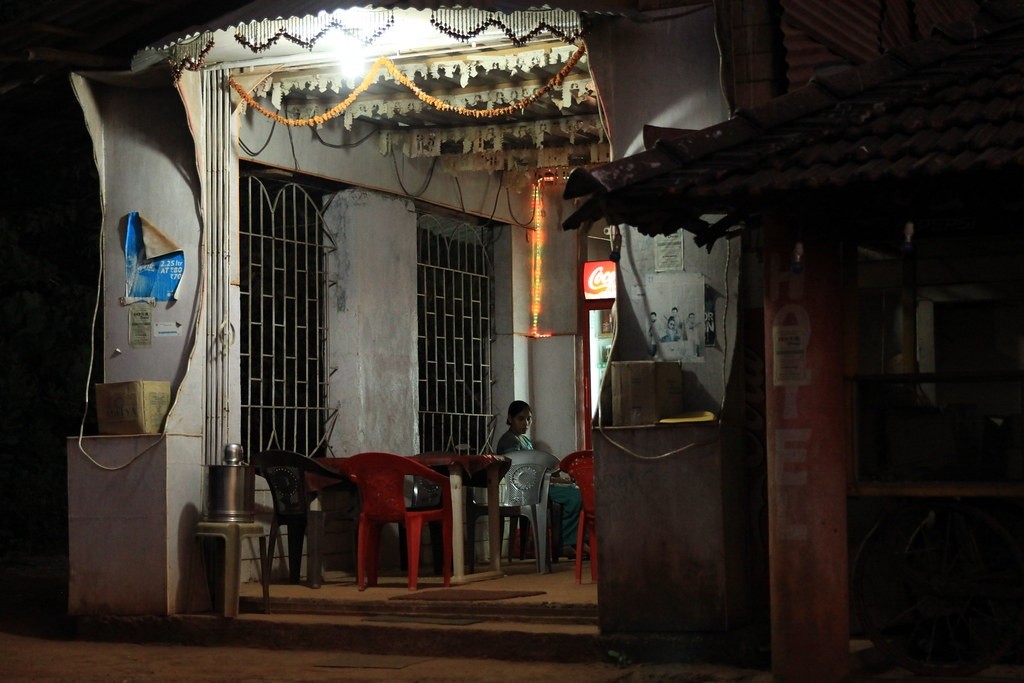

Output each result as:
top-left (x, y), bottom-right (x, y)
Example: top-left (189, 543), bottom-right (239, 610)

top-left (583, 260), bottom-right (616, 451)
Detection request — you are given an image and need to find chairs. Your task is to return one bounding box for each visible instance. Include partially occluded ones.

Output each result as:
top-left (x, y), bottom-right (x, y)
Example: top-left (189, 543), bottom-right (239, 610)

top-left (237, 441), bottom-right (600, 599)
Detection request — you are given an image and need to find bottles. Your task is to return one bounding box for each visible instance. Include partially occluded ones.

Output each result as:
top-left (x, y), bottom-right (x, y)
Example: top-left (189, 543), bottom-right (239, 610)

top-left (603, 311), bottom-right (610, 333)
top-left (605, 345), bottom-right (611, 362)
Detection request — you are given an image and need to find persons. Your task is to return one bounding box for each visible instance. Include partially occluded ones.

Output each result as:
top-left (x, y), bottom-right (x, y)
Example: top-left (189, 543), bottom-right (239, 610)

top-left (497, 400), bottom-right (590, 560)
top-left (647, 307), bottom-right (702, 357)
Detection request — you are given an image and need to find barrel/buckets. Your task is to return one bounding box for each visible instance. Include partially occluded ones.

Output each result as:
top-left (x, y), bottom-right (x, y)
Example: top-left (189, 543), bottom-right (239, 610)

top-left (202, 444), bottom-right (257, 521)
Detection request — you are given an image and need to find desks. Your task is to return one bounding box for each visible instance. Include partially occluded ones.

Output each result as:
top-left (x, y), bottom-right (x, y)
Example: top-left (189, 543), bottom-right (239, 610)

top-left (389, 454), bottom-right (513, 584)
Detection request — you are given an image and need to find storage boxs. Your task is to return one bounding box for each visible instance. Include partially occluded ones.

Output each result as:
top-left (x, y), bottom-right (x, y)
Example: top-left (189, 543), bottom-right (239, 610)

top-left (95, 380), bottom-right (172, 435)
